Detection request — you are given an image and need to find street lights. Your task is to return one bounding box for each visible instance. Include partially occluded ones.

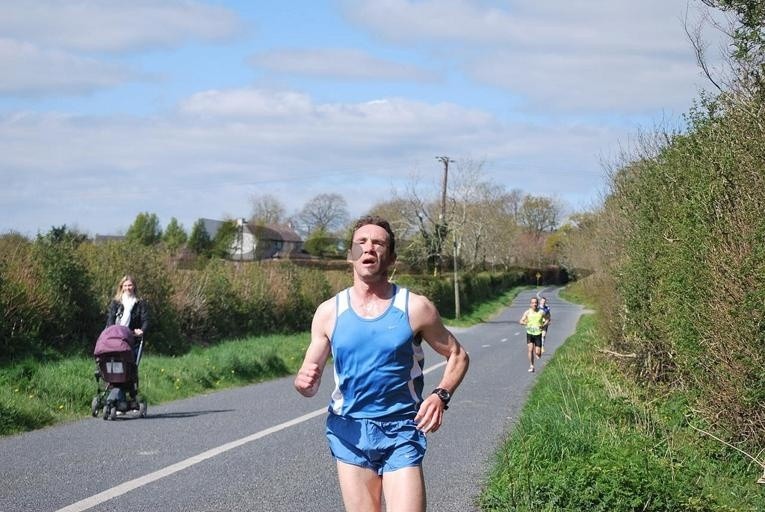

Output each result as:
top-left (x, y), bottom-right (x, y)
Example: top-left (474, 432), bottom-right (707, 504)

top-left (435, 154), bottom-right (456, 223)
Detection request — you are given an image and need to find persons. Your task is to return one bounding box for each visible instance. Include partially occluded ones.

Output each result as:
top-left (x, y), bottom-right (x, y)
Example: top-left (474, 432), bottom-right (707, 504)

top-left (539, 297), bottom-right (552, 354)
top-left (104, 273), bottom-right (149, 397)
top-left (519, 297), bottom-right (550, 372)
top-left (293, 217), bottom-right (470, 511)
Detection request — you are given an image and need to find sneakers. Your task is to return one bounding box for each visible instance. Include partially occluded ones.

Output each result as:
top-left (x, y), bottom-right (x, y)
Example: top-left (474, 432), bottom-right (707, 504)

top-left (528, 365), bottom-right (535, 373)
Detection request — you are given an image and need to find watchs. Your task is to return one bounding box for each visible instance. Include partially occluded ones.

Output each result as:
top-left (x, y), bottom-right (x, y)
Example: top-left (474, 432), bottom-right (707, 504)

top-left (431, 387), bottom-right (452, 410)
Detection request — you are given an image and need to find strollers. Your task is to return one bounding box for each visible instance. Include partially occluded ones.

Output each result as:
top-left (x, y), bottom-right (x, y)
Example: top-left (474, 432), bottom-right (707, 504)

top-left (87, 324), bottom-right (146, 425)
